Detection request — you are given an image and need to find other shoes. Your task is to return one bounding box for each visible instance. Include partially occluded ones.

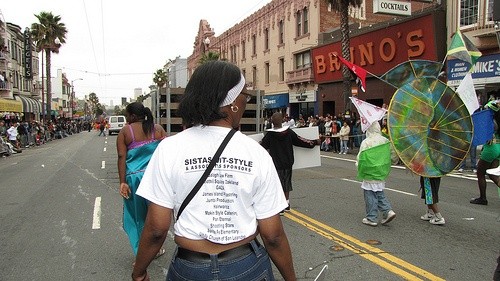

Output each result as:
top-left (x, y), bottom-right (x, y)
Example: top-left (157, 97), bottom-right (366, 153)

top-left (279, 204), bottom-right (290, 216)
top-left (154, 248), bottom-right (166, 258)
top-left (470, 198), bottom-right (487, 205)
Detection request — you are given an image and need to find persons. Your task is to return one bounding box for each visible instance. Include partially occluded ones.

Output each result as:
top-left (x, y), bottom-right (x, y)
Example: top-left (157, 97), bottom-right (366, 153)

top-left (458, 95), bottom-right (500, 205)
top-left (265, 111), bottom-right (350, 155)
top-left (353, 103), bottom-right (396, 226)
top-left (418, 175), bottom-right (445, 225)
top-left (0, 118), bottom-right (54, 158)
top-left (49, 119), bottom-right (107, 139)
top-left (116, 102), bottom-right (165, 274)
top-left (258, 112), bottom-right (321, 215)
top-left (132, 58), bottom-right (295, 281)
top-left (0, 43), bottom-right (13, 83)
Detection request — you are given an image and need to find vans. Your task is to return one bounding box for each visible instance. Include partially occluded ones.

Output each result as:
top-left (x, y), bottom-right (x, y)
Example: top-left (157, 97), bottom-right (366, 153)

top-left (108, 116), bottom-right (128, 135)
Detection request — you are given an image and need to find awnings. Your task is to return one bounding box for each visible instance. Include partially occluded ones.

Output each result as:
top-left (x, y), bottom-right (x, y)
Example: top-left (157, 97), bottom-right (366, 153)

top-left (0, 95), bottom-right (42, 113)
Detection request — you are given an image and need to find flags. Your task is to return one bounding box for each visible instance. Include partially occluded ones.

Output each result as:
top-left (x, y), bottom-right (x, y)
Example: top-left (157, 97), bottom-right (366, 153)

top-left (456, 70), bottom-right (479, 116)
top-left (447, 29), bottom-right (482, 66)
top-left (349, 97), bottom-right (387, 133)
top-left (470, 109), bottom-right (496, 146)
top-left (337, 55), bottom-right (367, 92)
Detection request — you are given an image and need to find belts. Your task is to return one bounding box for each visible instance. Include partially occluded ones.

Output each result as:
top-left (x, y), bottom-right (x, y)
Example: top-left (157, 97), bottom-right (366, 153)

top-left (174, 236), bottom-right (261, 264)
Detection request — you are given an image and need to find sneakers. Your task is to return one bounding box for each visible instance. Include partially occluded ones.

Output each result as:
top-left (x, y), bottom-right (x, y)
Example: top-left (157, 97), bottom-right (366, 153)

top-left (380, 210), bottom-right (396, 225)
top-left (362, 217), bottom-right (378, 226)
top-left (420, 213), bottom-right (434, 220)
top-left (429, 215), bottom-right (445, 225)
